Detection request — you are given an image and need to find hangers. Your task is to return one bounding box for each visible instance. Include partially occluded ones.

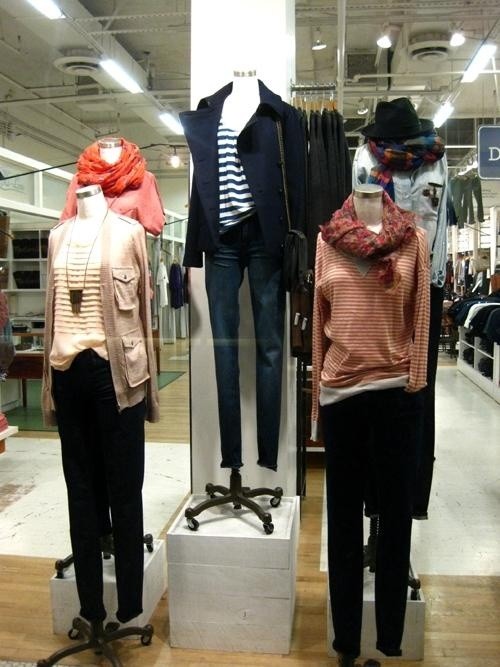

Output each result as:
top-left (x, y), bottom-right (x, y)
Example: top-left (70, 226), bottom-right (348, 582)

top-left (291, 81), bottom-right (344, 121)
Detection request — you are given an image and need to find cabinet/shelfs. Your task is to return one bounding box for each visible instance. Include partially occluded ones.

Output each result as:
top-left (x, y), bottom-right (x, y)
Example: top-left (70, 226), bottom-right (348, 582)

top-left (456, 324), bottom-right (500, 404)
top-left (1, 223), bottom-right (54, 298)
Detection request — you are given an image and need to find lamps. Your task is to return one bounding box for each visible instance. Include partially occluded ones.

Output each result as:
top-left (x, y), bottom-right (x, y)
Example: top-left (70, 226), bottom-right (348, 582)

top-left (449, 21), bottom-right (466, 47)
top-left (310, 26), bottom-right (328, 52)
top-left (375, 22), bottom-right (394, 50)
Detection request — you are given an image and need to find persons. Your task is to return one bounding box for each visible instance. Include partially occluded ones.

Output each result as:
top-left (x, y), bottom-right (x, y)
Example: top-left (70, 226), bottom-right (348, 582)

top-left (36, 182), bottom-right (160, 623)
top-left (62, 134), bottom-right (166, 235)
top-left (309, 181), bottom-right (429, 657)
top-left (348, 93), bottom-right (452, 514)
top-left (179, 61), bottom-right (302, 469)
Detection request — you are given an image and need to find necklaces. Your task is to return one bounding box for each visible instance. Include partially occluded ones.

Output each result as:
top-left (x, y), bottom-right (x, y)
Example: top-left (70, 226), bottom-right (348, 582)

top-left (65, 212), bottom-right (107, 313)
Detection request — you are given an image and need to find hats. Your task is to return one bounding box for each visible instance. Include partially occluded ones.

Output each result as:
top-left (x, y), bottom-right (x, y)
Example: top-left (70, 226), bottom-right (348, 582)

top-left (360, 96), bottom-right (434, 140)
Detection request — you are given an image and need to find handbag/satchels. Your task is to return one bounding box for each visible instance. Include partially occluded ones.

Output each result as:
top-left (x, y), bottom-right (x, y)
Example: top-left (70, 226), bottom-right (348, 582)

top-left (277, 226), bottom-right (312, 294)
